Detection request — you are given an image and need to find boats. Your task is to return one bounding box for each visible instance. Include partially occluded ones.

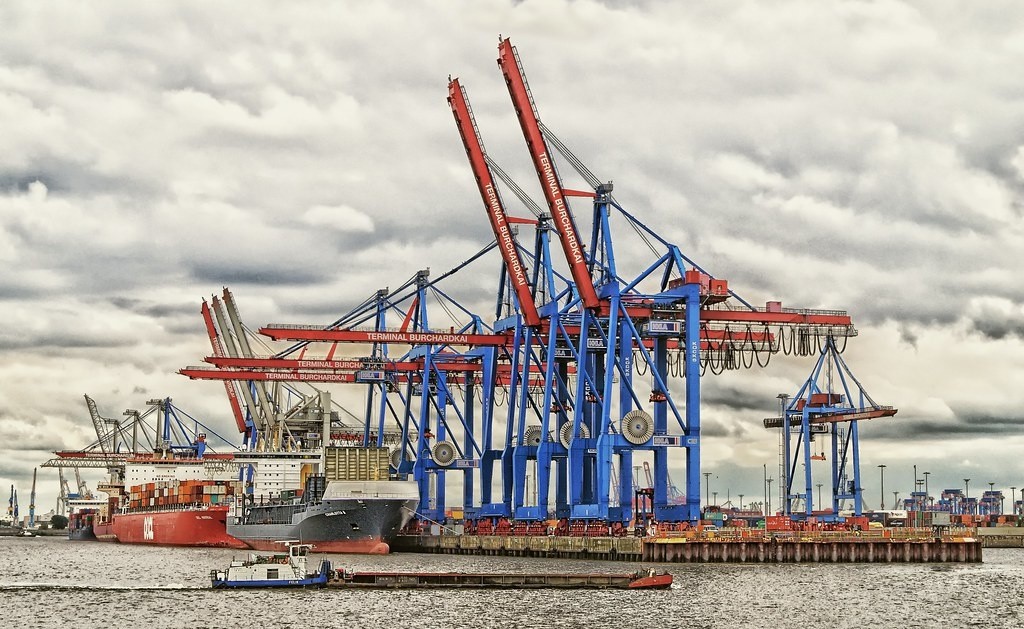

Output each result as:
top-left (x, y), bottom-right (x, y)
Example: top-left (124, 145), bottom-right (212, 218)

top-left (67, 462), bottom-right (421, 554)
top-left (207, 539), bottom-right (332, 589)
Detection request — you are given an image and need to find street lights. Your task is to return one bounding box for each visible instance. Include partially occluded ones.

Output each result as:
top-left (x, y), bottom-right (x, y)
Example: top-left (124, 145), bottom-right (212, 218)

top-left (702, 472), bottom-right (712, 506)
top-left (964, 478), bottom-right (971, 509)
top-left (878, 464), bottom-right (887, 510)
top-left (816, 483), bottom-right (823, 510)
top-left (767, 478), bottom-right (774, 516)
top-left (893, 491), bottom-right (899, 508)
top-left (923, 472), bottom-right (930, 505)
top-left (989, 482), bottom-right (995, 514)
top-left (916, 479), bottom-right (925, 511)
top-left (712, 491), bottom-right (718, 506)
top-left (738, 494), bottom-right (744, 511)
top-left (1010, 486), bottom-right (1016, 514)
